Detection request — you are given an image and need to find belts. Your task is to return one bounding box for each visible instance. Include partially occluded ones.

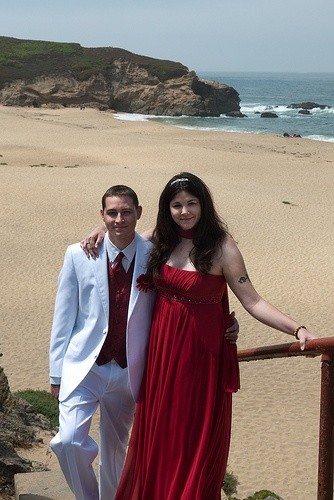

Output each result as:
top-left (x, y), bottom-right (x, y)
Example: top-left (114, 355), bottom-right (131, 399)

top-left (111, 252), bottom-right (126, 286)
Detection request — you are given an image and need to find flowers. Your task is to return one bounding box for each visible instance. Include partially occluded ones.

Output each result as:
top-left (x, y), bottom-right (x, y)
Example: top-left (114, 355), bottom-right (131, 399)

top-left (136, 274), bottom-right (155, 293)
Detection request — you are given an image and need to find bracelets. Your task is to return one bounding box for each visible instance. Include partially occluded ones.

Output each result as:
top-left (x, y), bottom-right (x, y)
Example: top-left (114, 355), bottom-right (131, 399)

top-left (295, 325), bottom-right (307, 340)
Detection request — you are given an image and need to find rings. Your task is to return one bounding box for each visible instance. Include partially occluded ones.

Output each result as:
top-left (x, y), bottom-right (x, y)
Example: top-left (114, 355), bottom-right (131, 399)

top-left (86, 242), bottom-right (90, 245)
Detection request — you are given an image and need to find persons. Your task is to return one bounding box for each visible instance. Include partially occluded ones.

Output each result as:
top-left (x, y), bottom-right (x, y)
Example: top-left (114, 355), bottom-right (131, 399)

top-left (49, 185), bottom-right (239, 499)
top-left (79, 173), bottom-right (317, 499)
